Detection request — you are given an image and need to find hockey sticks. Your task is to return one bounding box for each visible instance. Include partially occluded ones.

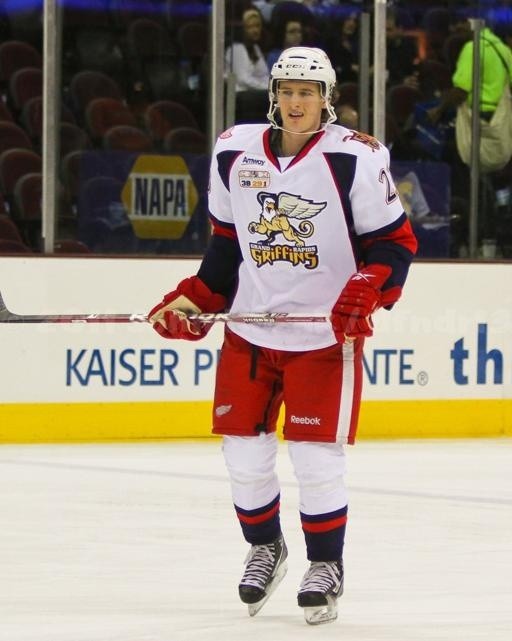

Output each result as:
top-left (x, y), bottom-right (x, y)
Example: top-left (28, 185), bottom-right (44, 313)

top-left (0, 290), bottom-right (332, 326)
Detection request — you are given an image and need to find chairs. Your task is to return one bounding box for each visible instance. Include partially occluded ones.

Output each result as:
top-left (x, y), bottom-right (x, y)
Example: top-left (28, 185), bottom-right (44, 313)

top-left (0, 8), bottom-right (511, 259)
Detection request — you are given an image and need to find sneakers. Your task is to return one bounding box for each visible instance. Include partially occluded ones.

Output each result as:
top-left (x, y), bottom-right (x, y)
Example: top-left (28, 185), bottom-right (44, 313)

top-left (297, 561), bottom-right (344, 607)
top-left (416, 211), bottom-right (445, 224)
top-left (239, 531), bottom-right (287, 604)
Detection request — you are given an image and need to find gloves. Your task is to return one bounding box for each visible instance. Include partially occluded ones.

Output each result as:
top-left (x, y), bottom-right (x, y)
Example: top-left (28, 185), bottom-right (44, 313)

top-left (330, 264), bottom-right (401, 344)
top-left (148, 275), bottom-right (228, 341)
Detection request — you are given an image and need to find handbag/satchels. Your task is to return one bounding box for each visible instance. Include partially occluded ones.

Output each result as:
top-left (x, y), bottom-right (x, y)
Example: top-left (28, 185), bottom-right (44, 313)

top-left (455, 81), bottom-right (511, 173)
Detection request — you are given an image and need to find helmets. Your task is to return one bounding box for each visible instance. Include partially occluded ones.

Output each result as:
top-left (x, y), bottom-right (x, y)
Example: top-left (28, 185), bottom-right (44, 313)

top-left (268, 48), bottom-right (337, 108)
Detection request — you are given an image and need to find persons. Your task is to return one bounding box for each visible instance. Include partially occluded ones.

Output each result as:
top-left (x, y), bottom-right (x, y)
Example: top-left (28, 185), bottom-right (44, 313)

top-left (144, 46), bottom-right (420, 609)
top-left (223, 3), bottom-right (512, 259)
